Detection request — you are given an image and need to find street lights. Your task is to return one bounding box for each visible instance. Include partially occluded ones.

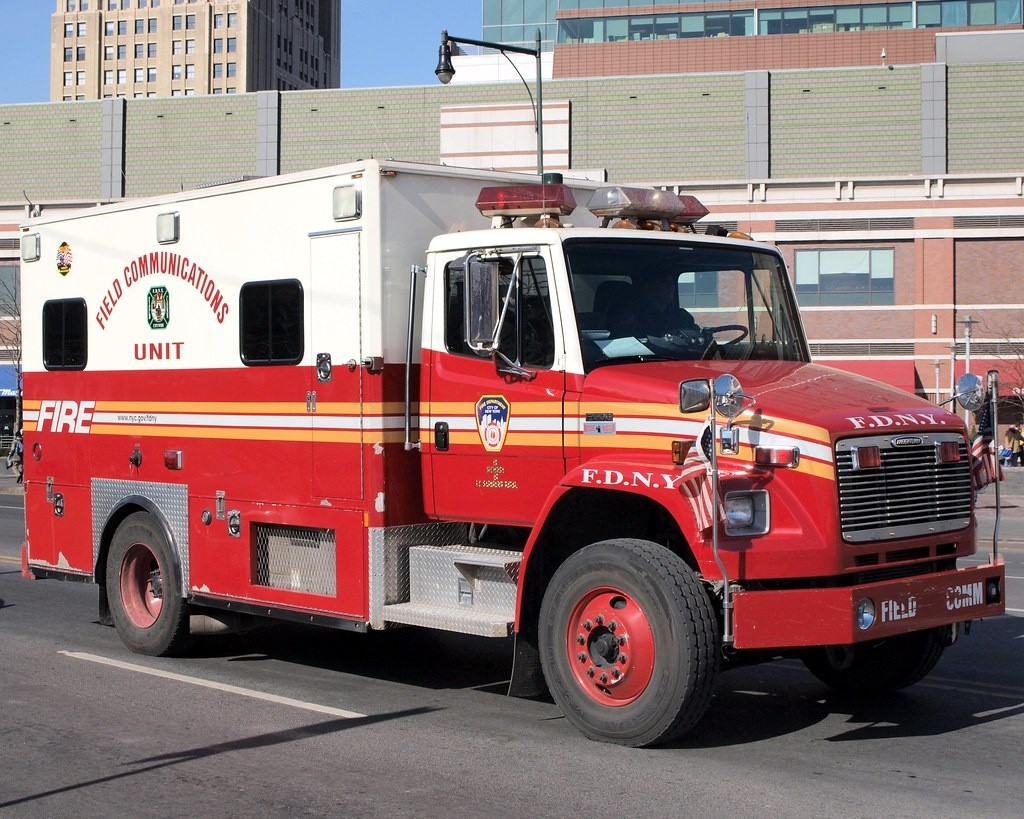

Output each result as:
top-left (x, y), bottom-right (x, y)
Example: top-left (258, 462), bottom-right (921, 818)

top-left (930, 361), bottom-right (946, 405)
top-left (435, 30), bottom-right (544, 175)
top-left (955, 315), bottom-right (982, 430)
top-left (944, 344), bottom-right (960, 412)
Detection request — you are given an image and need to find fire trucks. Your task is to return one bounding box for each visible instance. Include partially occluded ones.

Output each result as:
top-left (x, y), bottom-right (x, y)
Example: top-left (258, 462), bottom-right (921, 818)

top-left (18, 158), bottom-right (1008, 747)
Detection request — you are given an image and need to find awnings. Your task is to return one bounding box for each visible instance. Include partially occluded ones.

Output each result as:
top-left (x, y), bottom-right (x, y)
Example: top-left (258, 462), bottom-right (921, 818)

top-left (812, 360), bottom-right (915, 394)
top-left (0, 364), bottom-right (23, 397)
top-left (954, 359), bottom-right (1024, 396)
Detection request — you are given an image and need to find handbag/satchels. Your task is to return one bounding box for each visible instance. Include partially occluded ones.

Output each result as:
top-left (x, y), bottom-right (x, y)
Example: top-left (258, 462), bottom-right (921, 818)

top-left (6, 455), bottom-right (13, 469)
top-left (1002, 447), bottom-right (1013, 457)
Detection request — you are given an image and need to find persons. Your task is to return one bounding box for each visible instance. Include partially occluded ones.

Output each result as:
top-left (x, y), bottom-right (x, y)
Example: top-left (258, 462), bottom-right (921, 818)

top-left (1005, 422), bottom-right (1024, 468)
top-left (607, 261), bottom-right (702, 359)
top-left (7, 431), bottom-right (24, 483)
top-left (473, 263), bottom-right (554, 370)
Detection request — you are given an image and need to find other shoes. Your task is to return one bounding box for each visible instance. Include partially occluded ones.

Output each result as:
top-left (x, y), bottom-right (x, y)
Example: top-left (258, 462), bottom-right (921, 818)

top-left (17, 474), bottom-right (22, 483)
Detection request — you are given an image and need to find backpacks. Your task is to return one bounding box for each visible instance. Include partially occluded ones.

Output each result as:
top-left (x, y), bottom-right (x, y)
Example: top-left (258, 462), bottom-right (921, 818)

top-left (14, 438), bottom-right (23, 455)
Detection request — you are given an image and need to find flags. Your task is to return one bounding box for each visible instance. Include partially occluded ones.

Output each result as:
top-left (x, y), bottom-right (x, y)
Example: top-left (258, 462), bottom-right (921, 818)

top-left (971, 380), bottom-right (1005, 491)
top-left (669, 418), bottom-right (728, 534)
